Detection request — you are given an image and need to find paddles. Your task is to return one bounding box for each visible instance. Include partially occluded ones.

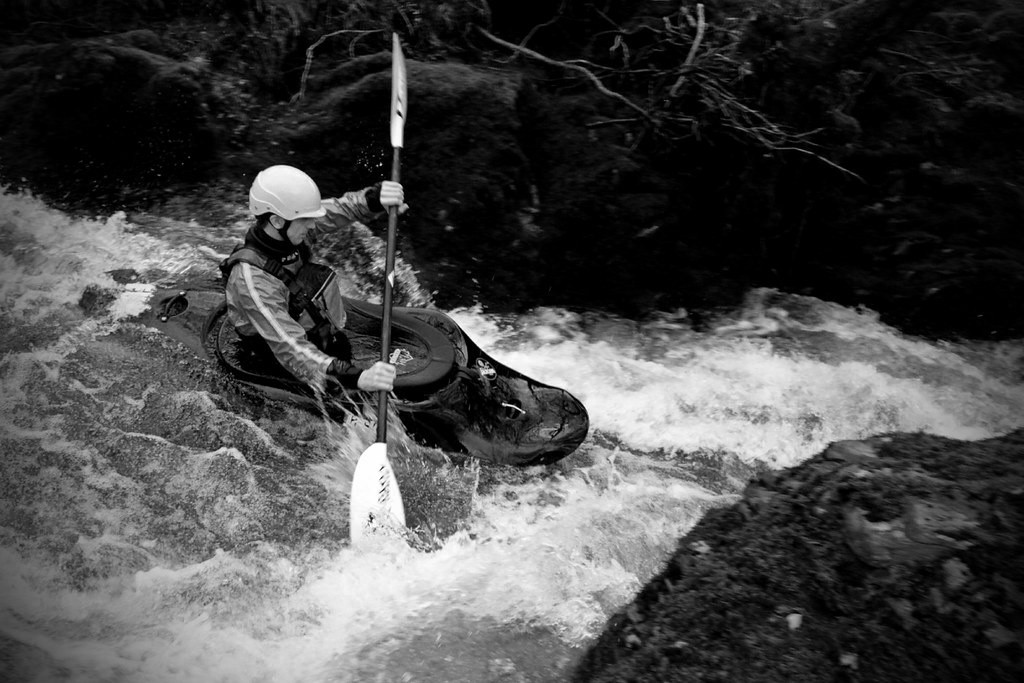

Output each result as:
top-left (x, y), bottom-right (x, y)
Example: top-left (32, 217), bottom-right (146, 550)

top-left (348, 28), bottom-right (412, 560)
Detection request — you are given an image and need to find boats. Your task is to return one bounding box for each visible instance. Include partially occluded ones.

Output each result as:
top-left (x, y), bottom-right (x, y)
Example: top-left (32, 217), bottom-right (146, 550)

top-left (81, 270), bottom-right (589, 469)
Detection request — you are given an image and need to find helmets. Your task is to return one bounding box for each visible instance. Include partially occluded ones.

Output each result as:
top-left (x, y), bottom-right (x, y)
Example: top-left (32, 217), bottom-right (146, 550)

top-left (249, 165), bottom-right (326, 220)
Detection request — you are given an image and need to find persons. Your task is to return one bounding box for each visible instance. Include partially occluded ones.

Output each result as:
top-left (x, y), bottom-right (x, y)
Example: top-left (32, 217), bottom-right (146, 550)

top-left (219, 164), bottom-right (408, 401)
